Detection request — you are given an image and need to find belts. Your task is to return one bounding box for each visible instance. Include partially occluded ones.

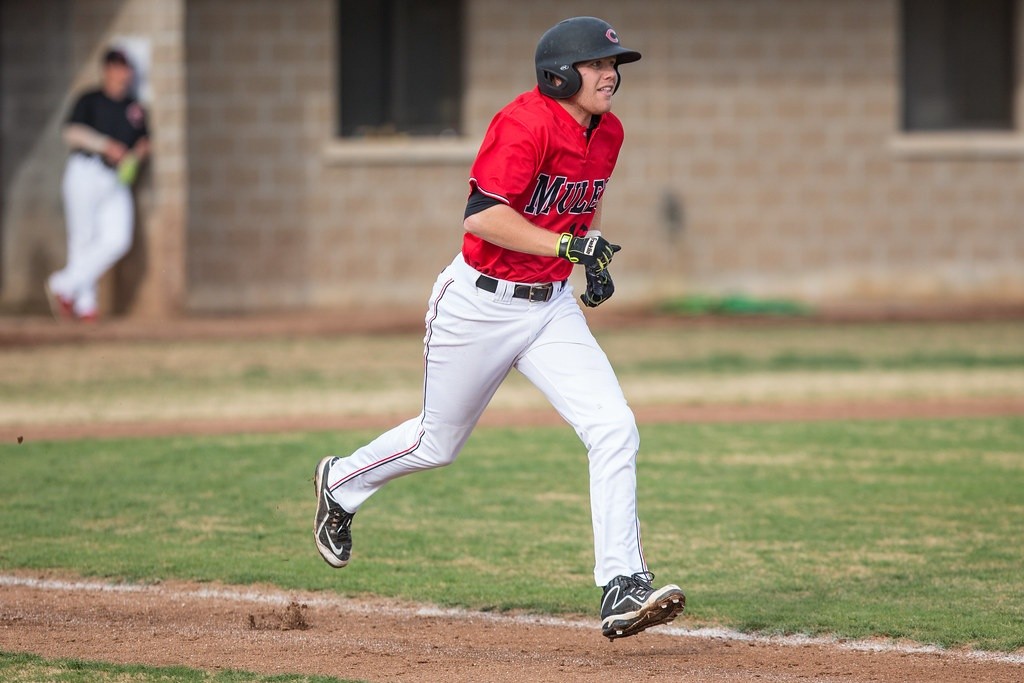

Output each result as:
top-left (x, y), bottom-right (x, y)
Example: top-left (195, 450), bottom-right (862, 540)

top-left (476, 275), bottom-right (568, 302)
top-left (74, 148), bottom-right (116, 169)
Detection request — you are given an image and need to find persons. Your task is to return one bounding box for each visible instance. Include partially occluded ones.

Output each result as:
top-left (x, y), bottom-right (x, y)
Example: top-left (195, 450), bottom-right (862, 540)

top-left (44, 51), bottom-right (150, 321)
top-left (313, 17), bottom-right (686, 642)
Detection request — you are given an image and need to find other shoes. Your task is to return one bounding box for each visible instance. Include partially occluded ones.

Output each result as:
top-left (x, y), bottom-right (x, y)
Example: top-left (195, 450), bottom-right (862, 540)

top-left (77, 307), bottom-right (98, 323)
top-left (45, 278), bottom-right (72, 324)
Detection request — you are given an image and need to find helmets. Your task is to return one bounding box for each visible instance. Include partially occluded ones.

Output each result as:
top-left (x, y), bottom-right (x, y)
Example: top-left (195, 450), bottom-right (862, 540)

top-left (535, 16), bottom-right (641, 99)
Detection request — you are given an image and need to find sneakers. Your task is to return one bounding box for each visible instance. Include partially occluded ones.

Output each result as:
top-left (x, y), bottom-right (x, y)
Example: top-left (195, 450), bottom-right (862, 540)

top-left (314, 456), bottom-right (352, 568)
top-left (601, 571), bottom-right (686, 642)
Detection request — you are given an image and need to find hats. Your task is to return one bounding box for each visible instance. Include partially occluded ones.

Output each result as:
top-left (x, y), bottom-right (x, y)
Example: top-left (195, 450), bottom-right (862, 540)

top-left (102, 50), bottom-right (135, 67)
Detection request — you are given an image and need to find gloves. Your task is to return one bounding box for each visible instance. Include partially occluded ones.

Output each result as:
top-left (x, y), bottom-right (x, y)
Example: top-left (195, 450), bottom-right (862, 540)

top-left (580, 264), bottom-right (614, 308)
top-left (556, 232), bottom-right (622, 269)
